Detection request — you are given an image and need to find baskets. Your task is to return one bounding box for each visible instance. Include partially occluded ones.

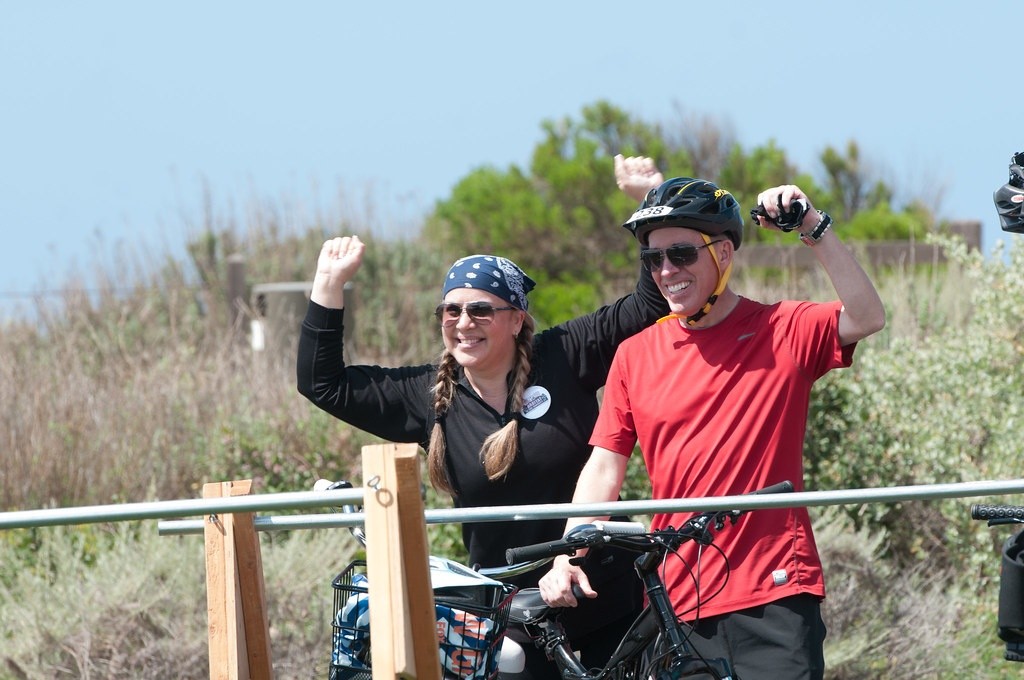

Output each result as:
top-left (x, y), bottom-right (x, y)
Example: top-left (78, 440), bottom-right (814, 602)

top-left (329, 560), bottom-right (518, 680)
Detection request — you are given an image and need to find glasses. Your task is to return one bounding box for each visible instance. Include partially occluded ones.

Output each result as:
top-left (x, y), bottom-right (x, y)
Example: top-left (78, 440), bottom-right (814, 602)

top-left (639, 239), bottom-right (724, 271)
top-left (435, 302), bottom-right (514, 328)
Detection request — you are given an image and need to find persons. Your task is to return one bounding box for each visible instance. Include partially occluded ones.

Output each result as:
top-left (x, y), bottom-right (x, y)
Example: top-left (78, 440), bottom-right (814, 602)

top-left (297, 153), bottom-right (664, 680)
top-left (538, 177), bottom-right (886, 680)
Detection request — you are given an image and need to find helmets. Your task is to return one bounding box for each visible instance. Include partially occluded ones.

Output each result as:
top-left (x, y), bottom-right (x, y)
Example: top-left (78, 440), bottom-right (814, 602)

top-left (623, 177), bottom-right (744, 252)
top-left (993, 150), bottom-right (1024, 233)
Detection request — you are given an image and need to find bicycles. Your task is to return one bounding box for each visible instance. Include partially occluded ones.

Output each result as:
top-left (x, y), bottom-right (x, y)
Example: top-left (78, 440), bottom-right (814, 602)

top-left (310, 479), bottom-right (794, 680)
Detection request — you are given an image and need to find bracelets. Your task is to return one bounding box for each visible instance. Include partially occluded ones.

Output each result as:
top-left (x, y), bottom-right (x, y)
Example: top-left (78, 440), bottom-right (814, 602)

top-left (800, 210), bottom-right (833, 247)
